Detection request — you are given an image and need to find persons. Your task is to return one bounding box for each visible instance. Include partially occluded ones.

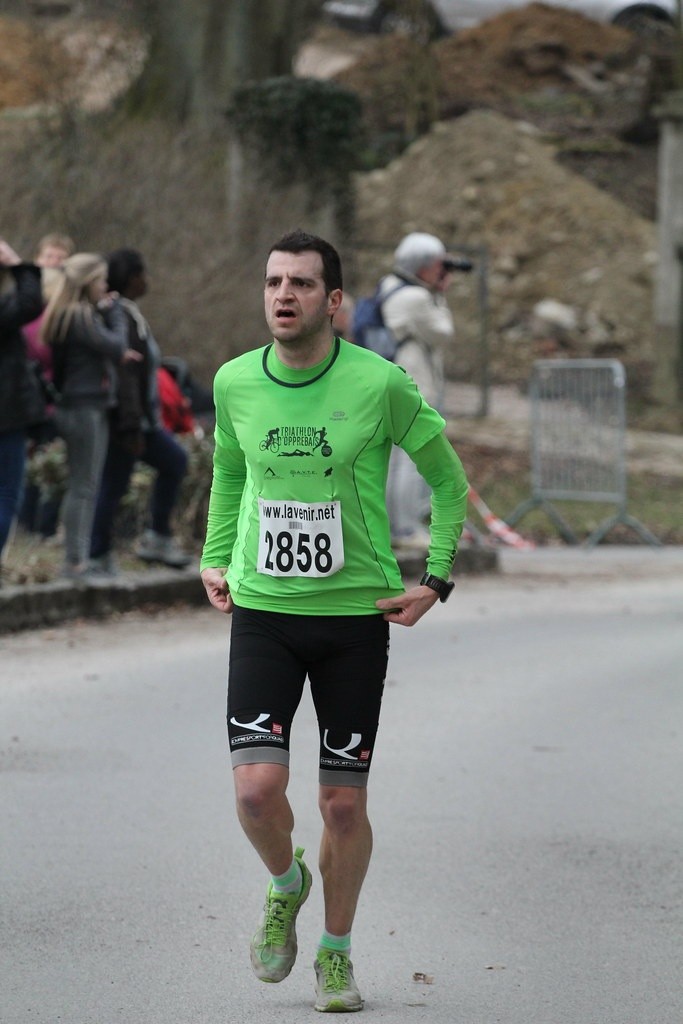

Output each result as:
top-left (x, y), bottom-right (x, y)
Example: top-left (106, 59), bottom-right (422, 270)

top-left (377, 232), bottom-right (455, 551)
top-left (0, 233), bottom-right (188, 575)
top-left (332, 289), bottom-right (356, 345)
top-left (200, 228), bottom-right (470, 1012)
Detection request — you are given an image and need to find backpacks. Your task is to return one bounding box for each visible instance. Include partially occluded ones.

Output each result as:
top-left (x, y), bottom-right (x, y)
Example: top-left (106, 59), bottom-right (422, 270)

top-left (351, 274), bottom-right (411, 361)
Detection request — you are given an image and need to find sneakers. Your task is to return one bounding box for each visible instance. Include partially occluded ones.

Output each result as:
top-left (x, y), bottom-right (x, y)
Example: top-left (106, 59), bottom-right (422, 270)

top-left (249, 846), bottom-right (312, 983)
top-left (314, 945), bottom-right (365, 1011)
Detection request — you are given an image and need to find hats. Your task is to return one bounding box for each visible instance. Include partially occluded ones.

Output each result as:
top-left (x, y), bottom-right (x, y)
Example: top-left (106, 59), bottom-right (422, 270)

top-left (62, 253), bottom-right (107, 286)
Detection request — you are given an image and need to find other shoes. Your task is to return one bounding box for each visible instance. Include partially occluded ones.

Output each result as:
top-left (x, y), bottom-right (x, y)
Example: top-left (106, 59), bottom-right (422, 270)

top-left (133, 529), bottom-right (192, 565)
top-left (89, 551), bottom-right (118, 575)
top-left (59, 567), bottom-right (111, 588)
top-left (390, 522), bottom-right (430, 551)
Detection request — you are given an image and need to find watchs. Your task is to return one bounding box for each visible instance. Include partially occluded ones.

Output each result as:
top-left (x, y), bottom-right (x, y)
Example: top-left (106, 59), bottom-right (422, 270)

top-left (420, 573), bottom-right (455, 603)
